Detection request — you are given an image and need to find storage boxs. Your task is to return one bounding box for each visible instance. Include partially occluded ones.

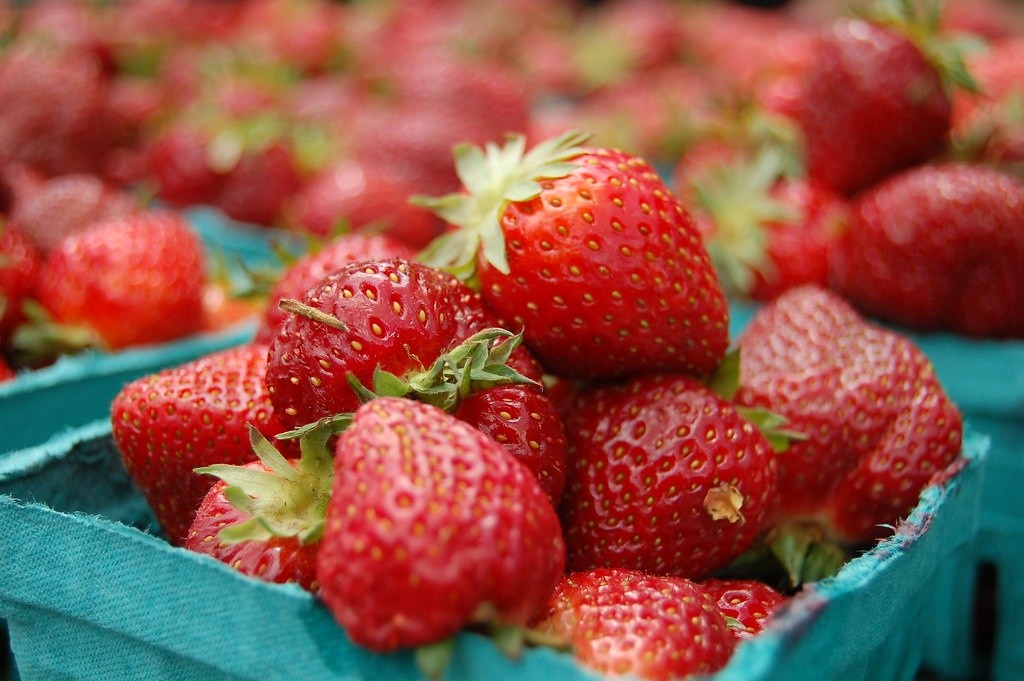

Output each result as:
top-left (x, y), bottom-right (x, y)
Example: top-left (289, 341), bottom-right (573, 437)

top-left (0, 204), bottom-right (1024, 681)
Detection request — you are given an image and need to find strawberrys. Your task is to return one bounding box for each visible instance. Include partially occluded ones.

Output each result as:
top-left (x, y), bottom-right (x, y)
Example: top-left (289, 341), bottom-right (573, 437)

top-left (0, 0), bottom-right (1024, 681)
